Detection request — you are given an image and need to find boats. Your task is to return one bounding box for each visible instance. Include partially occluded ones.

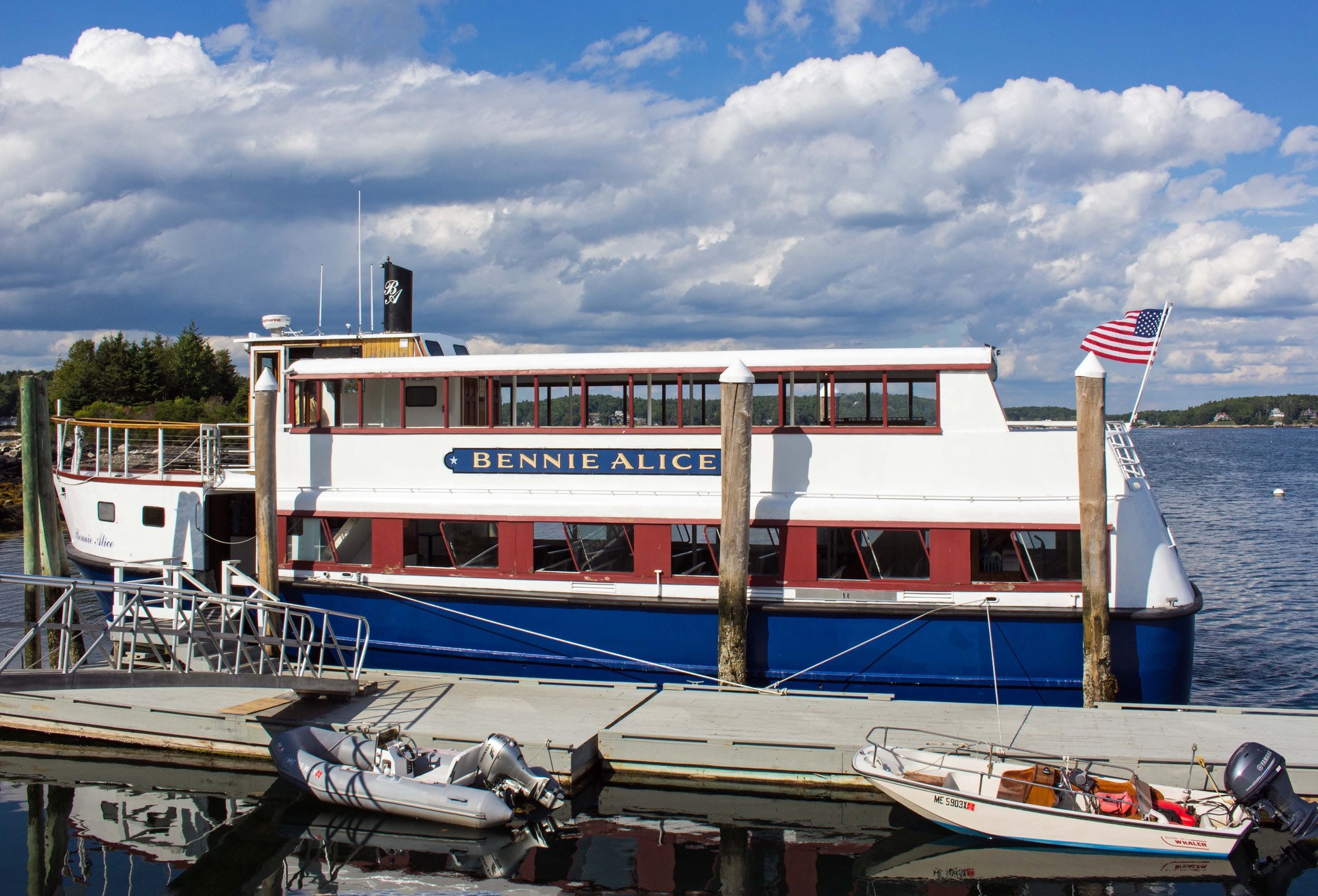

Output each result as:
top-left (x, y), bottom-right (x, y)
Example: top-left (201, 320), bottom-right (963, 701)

top-left (267, 723), bottom-right (569, 833)
top-left (850, 812), bottom-right (1318, 895)
top-left (849, 722), bottom-right (1318, 864)
top-left (49, 167), bottom-right (1206, 707)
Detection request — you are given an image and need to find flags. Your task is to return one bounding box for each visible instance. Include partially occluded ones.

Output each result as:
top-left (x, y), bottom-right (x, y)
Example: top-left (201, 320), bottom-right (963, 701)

top-left (1080, 308), bottom-right (1169, 366)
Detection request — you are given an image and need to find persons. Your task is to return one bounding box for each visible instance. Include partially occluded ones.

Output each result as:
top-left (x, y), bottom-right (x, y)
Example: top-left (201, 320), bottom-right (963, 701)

top-left (294, 384), bottom-right (317, 427)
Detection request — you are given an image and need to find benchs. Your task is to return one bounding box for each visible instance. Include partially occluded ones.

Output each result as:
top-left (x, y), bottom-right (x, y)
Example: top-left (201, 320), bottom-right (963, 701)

top-left (462, 542), bottom-right (780, 576)
